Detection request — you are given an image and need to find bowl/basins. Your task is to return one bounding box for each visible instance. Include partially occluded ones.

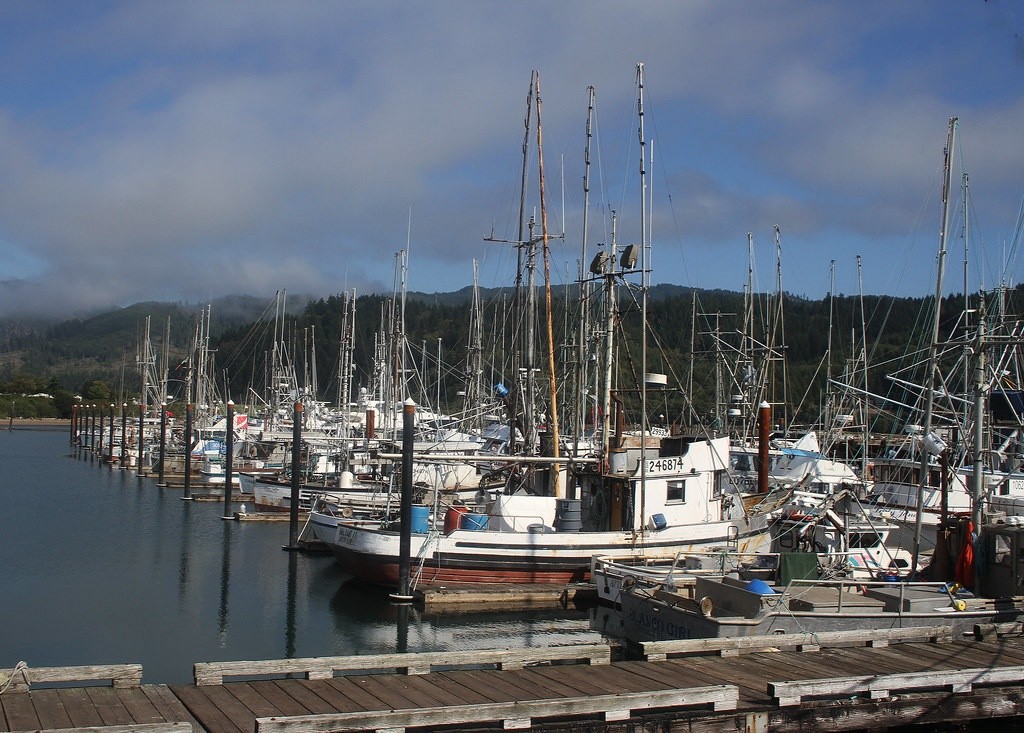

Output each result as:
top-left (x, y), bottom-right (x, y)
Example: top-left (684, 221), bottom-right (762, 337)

top-left (746, 579), bottom-right (775, 594)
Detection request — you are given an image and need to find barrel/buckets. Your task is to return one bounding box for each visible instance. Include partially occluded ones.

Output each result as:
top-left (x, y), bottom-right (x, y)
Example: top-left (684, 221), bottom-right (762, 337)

top-left (461, 513), bottom-right (489, 531)
top-left (443, 507), bottom-right (467, 535)
top-left (411, 506), bottom-right (429, 533)
top-left (552, 499), bottom-right (583, 532)
top-left (339, 471), bottom-right (354, 489)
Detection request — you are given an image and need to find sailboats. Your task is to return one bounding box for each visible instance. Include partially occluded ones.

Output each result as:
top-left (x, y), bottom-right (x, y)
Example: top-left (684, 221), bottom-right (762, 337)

top-left (87, 62), bottom-right (1024, 645)
